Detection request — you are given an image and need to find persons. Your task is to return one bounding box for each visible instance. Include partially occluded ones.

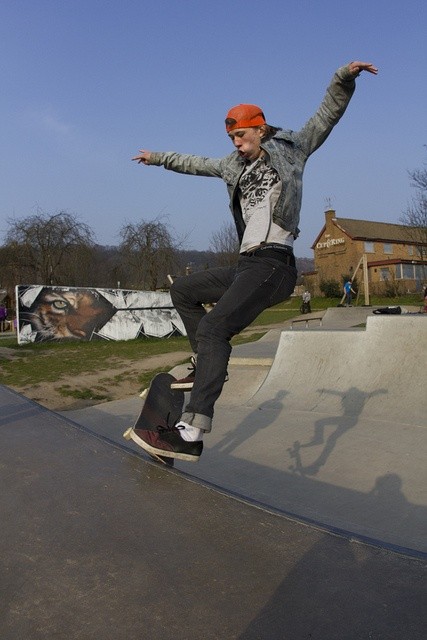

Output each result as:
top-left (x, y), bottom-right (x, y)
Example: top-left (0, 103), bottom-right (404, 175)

top-left (128, 58), bottom-right (382, 464)
top-left (344, 280), bottom-right (356, 307)
top-left (302, 290), bottom-right (311, 313)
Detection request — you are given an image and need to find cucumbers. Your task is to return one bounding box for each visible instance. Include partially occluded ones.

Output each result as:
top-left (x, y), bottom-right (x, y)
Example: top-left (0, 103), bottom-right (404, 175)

top-left (122, 372), bottom-right (185, 467)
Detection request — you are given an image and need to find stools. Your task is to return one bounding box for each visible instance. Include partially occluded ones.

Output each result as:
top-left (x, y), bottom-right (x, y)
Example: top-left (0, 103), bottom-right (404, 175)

top-left (241, 249), bottom-right (296, 266)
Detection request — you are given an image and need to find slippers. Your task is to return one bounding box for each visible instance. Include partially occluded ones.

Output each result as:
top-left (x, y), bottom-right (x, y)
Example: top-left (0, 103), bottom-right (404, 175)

top-left (373, 306), bottom-right (400, 314)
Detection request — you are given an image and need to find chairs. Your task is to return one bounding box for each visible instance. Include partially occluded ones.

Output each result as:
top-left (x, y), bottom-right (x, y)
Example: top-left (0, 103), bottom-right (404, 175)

top-left (170, 356), bottom-right (229, 389)
top-left (130, 412), bottom-right (204, 462)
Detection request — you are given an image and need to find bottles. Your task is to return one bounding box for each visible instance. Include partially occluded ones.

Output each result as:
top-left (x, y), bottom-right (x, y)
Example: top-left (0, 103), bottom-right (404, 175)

top-left (226, 104), bottom-right (283, 132)
